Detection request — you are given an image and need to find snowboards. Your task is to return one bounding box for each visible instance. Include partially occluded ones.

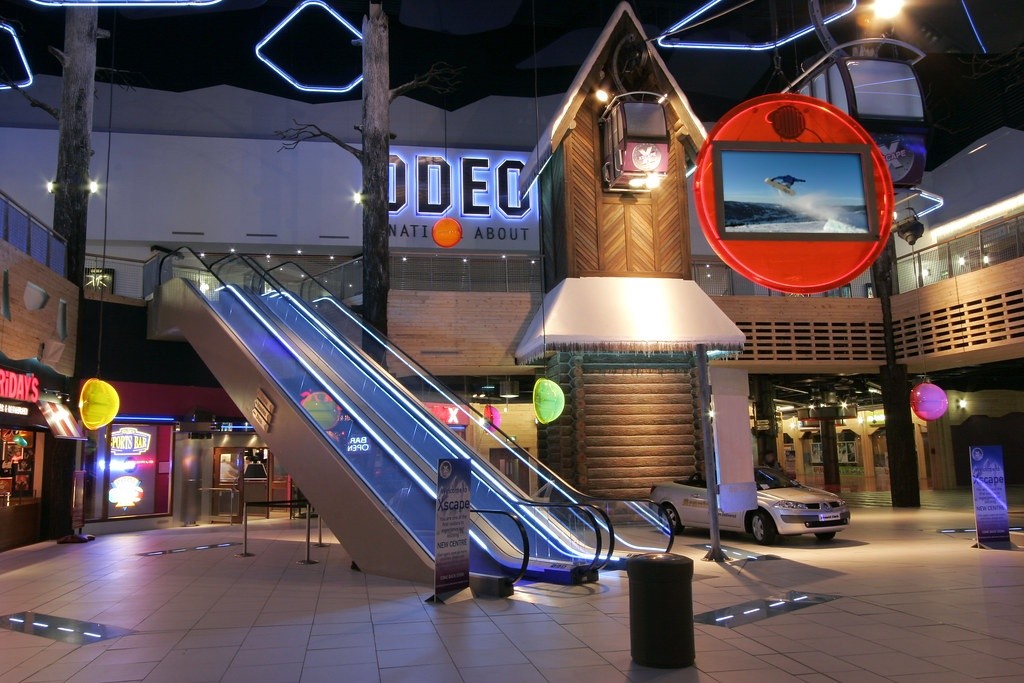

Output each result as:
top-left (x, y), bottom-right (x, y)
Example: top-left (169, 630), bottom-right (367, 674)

top-left (764, 178), bottom-right (796, 196)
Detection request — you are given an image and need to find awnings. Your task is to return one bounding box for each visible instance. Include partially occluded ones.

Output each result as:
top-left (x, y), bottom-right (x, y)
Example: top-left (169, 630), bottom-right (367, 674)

top-left (35, 400), bottom-right (88, 441)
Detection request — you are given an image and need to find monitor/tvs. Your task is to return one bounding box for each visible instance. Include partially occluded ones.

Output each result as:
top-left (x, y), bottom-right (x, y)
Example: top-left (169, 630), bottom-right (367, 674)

top-left (711, 139), bottom-right (880, 242)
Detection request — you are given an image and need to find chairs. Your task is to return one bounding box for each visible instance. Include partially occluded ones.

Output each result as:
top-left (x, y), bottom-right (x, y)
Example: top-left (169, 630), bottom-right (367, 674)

top-left (688, 472), bottom-right (702, 488)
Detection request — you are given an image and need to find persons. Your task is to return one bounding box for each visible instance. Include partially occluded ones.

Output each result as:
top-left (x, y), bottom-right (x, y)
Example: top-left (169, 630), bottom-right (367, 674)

top-left (771, 174), bottom-right (807, 190)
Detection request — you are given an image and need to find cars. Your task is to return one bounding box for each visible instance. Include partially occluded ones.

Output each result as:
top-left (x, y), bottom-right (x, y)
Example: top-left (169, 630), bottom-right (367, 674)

top-left (649, 466), bottom-right (851, 543)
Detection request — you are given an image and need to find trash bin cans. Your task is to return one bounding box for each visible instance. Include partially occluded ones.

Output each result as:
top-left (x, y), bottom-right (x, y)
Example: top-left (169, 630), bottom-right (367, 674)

top-left (625, 550), bottom-right (699, 670)
